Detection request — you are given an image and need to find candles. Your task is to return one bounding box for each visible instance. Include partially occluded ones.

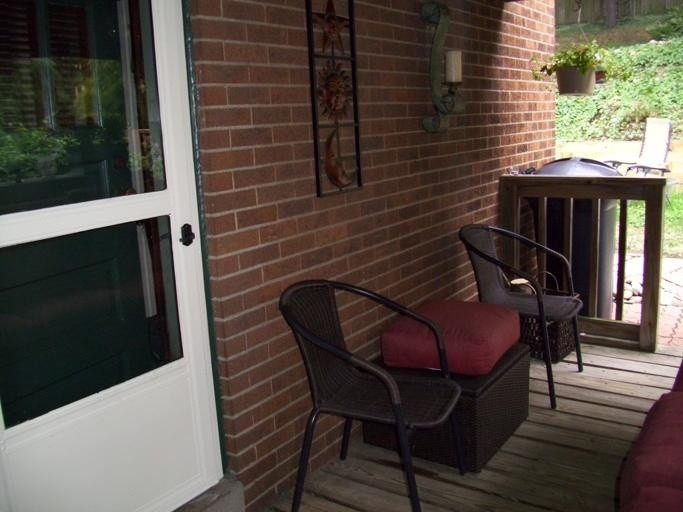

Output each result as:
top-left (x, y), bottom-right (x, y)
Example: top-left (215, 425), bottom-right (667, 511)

top-left (444, 47), bottom-right (463, 83)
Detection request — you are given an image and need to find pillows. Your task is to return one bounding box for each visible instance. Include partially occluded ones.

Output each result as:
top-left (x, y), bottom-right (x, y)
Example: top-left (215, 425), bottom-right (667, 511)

top-left (381, 298), bottom-right (521, 376)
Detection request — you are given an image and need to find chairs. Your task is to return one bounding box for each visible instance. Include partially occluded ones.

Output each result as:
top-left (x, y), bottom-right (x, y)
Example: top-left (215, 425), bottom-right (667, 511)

top-left (602, 116), bottom-right (678, 212)
top-left (458, 222), bottom-right (583, 412)
top-left (279, 277), bottom-right (472, 512)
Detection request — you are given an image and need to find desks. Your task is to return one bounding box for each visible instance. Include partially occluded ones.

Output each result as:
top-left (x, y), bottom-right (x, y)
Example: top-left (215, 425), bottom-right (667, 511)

top-left (359, 344), bottom-right (535, 473)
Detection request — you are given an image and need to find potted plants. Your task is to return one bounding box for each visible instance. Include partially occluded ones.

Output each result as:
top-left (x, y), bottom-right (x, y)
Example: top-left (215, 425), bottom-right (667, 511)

top-left (528, 35), bottom-right (636, 94)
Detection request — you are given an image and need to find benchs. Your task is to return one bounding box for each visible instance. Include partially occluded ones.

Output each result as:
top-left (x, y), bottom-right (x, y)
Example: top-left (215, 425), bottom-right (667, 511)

top-left (614, 358), bottom-right (683, 512)
